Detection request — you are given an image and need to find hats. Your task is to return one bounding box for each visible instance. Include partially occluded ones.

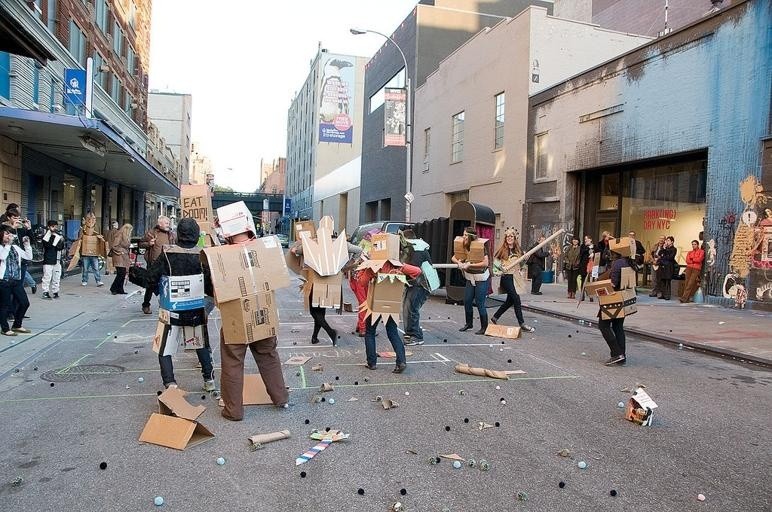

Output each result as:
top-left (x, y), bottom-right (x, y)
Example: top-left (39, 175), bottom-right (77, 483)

top-left (178, 218), bottom-right (199, 247)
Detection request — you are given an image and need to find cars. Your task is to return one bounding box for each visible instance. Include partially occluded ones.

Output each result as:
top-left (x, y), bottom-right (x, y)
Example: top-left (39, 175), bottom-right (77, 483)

top-left (273, 234), bottom-right (289, 248)
top-left (350, 221), bottom-right (415, 243)
top-left (127, 237), bottom-right (148, 256)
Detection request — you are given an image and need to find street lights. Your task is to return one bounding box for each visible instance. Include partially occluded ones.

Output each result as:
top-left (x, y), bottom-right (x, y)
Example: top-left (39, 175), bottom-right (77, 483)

top-left (348, 26), bottom-right (412, 221)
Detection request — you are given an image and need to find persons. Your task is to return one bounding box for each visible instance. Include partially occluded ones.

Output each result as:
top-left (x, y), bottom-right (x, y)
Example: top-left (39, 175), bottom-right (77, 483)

top-left (41, 211), bottom-right (134, 299)
top-left (491, 226), bottom-right (536, 332)
top-left (293, 217), bottom-right (433, 372)
top-left (561, 224), bottom-right (706, 367)
top-left (0, 202), bottom-right (37, 336)
top-left (524, 234), bottom-right (552, 296)
top-left (451, 226), bottom-right (491, 334)
top-left (126, 215), bottom-right (289, 421)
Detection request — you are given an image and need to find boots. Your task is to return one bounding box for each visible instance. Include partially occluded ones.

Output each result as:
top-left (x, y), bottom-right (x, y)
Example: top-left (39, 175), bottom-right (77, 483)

top-left (568, 289), bottom-right (575, 299)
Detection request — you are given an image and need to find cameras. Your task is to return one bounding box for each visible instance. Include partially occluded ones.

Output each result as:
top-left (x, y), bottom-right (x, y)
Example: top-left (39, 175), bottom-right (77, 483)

top-left (13, 235), bottom-right (18, 239)
top-left (22, 237), bottom-right (27, 242)
top-left (20, 219), bottom-right (27, 225)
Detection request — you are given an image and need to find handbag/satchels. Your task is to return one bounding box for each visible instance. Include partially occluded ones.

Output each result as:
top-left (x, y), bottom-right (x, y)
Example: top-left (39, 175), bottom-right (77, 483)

top-left (128, 266), bottom-right (147, 287)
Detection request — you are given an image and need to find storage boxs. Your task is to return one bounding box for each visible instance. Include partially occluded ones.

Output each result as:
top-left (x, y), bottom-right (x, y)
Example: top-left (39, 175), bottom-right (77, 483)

top-left (597, 288), bottom-right (638, 320)
top-left (356, 272), bottom-right (405, 328)
top-left (356, 233), bottom-right (402, 274)
top-left (217, 200), bottom-right (256, 239)
top-left (159, 273), bottom-right (206, 312)
top-left (180, 183), bottom-right (214, 224)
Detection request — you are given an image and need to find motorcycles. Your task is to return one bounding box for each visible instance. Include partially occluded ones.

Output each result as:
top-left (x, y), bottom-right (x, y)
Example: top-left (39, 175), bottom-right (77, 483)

top-left (30, 224), bottom-right (103, 272)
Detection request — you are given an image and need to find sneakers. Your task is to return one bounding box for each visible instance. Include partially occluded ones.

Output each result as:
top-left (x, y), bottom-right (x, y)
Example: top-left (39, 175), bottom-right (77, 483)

top-left (404, 333), bottom-right (424, 346)
top-left (165, 381), bottom-right (178, 389)
top-left (32, 284), bottom-right (60, 300)
top-left (491, 317), bottom-right (498, 325)
top-left (112, 289), bottom-right (128, 295)
top-left (81, 279), bottom-right (87, 286)
top-left (202, 381), bottom-right (216, 392)
top-left (521, 324), bottom-right (532, 331)
top-left (367, 363), bottom-right (377, 370)
top-left (531, 291), bottom-right (542, 295)
top-left (312, 337), bottom-right (319, 344)
top-left (96, 281), bottom-right (103, 287)
top-left (222, 409), bottom-right (242, 421)
top-left (680, 298), bottom-right (686, 302)
top-left (331, 330), bottom-right (336, 345)
top-left (393, 362), bottom-right (406, 373)
top-left (605, 354), bottom-right (626, 367)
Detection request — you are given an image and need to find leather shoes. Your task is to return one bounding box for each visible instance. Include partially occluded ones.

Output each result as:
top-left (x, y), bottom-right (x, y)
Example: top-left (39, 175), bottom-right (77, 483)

top-left (11, 327), bottom-right (31, 333)
top-left (476, 326), bottom-right (486, 334)
top-left (459, 324), bottom-right (473, 331)
top-left (1, 330), bottom-right (17, 336)
